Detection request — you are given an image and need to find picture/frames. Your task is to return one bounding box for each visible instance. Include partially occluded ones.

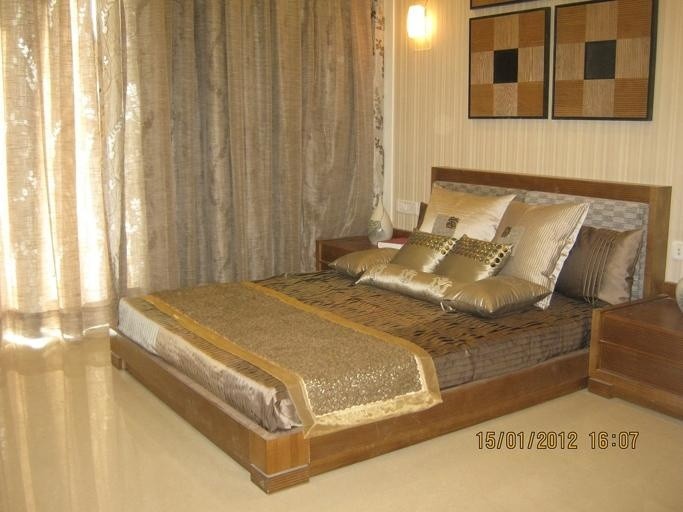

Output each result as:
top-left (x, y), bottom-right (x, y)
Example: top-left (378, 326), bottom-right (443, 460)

top-left (465, 6), bottom-right (551, 120)
top-left (551, 0), bottom-right (659, 123)
top-left (467, 0), bottom-right (543, 10)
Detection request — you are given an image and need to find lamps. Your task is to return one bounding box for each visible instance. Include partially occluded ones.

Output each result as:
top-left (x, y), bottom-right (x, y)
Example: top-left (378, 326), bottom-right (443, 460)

top-left (402, 2), bottom-right (440, 54)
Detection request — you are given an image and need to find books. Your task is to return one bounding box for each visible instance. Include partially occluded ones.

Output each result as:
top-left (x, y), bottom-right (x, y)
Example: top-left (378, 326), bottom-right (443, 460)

top-left (377, 237), bottom-right (408, 249)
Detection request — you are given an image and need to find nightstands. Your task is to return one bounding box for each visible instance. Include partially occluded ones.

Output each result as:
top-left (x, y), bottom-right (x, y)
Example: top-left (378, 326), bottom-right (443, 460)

top-left (586, 292), bottom-right (682, 422)
top-left (312, 234), bottom-right (373, 276)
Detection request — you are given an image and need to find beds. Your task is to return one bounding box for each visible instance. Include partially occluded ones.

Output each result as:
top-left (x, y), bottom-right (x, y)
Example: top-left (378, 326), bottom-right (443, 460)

top-left (108, 163), bottom-right (672, 495)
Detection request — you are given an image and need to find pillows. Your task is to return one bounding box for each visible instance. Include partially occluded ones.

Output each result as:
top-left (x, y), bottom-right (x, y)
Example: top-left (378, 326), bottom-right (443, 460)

top-left (323, 246), bottom-right (398, 279)
top-left (438, 271), bottom-right (554, 322)
top-left (548, 222), bottom-right (646, 313)
top-left (388, 228), bottom-right (459, 272)
top-left (432, 234), bottom-right (516, 282)
top-left (488, 190), bottom-right (593, 315)
top-left (417, 181), bottom-right (518, 248)
top-left (346, 259), bottom-right (477, 305)
top-left (415, 200), bottom-right (427, 231)
